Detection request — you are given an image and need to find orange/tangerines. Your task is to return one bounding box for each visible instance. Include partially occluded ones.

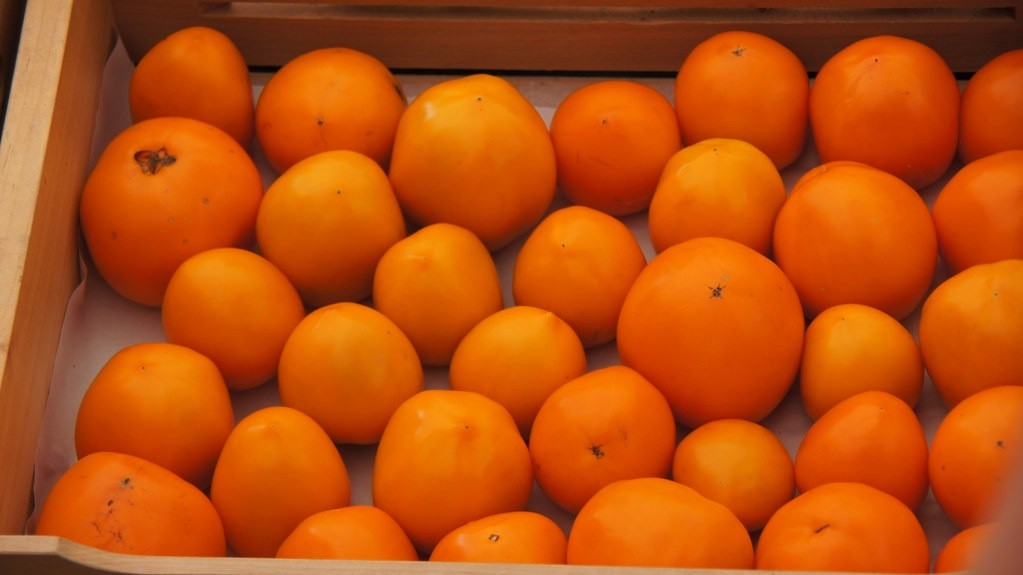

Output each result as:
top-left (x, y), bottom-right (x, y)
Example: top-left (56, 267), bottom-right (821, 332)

top-left (34, 27), bottom-right (1023, 572)
